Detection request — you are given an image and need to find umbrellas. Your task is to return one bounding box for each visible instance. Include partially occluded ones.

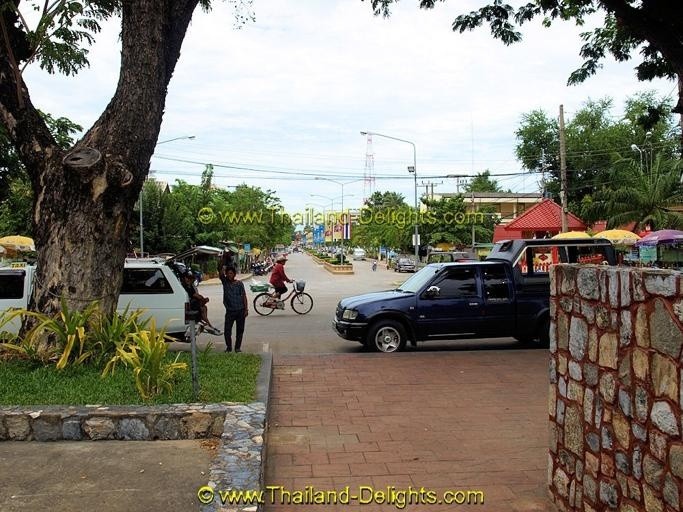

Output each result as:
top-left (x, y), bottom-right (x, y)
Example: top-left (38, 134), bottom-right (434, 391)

top-left (634, 228), bottom-right (683, 267)
top-left (550, 231), bottom-right (590, 240)
top-left (592, 228), bottom-right (640, 265)
top-left (0, 234), bottom-right (35, 263)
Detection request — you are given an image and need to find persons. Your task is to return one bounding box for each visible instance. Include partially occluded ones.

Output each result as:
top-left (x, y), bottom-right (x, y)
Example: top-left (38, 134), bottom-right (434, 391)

top-left (618, 252), bottom-right (622, 267)
top-left (218, 253), bottom-right (248, 353)
top-left (373, 260), bottom-right (377, 271)
top-left (269, 255), bottom-right (292, 310)
top-left (180, 272), bottom-right (225, 337)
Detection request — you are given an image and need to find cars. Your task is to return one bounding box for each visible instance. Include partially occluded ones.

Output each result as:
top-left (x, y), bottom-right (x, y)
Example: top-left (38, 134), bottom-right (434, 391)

top-left (0, 245), bottom-right (224, 343)
top-left (387, 258), bottom-right (416, 272)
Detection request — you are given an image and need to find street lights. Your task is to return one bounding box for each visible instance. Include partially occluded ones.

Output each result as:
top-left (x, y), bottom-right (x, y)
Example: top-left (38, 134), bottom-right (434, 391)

top-left (305, 176), bottom-right (371, 264)
top-left (360, 131), bottom-right (420, 272)
top-left (631, 132), bottom-right (653, 171)
top-left (138, 136), bottom-right (195, 258)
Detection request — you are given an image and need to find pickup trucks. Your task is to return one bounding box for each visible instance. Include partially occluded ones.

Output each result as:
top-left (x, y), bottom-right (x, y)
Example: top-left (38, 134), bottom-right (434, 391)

top-left (332, 261), bottom-right (549, 353)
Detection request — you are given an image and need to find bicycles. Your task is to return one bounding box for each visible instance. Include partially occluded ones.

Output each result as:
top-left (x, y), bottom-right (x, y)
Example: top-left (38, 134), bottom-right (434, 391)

top-left (253, 279), bottom-right (313, 316)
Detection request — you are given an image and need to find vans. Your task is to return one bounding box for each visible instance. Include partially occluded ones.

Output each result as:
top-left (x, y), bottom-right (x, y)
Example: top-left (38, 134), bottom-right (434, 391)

top-left (485, 239), bottom-right (618, 296)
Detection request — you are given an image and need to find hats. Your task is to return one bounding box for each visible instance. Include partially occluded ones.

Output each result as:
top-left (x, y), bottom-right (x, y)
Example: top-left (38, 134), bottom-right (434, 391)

top-left (276, 257), bottom-right (288, 263)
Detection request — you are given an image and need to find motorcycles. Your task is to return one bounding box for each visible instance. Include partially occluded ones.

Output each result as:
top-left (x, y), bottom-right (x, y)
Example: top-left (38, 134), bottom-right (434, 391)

top-left (252, 257), bottom-right (282, 276)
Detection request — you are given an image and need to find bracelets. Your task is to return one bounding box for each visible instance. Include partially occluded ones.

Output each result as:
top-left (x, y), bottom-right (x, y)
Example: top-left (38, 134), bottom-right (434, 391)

top-left (244, 309), bottom-right (248, 311)
